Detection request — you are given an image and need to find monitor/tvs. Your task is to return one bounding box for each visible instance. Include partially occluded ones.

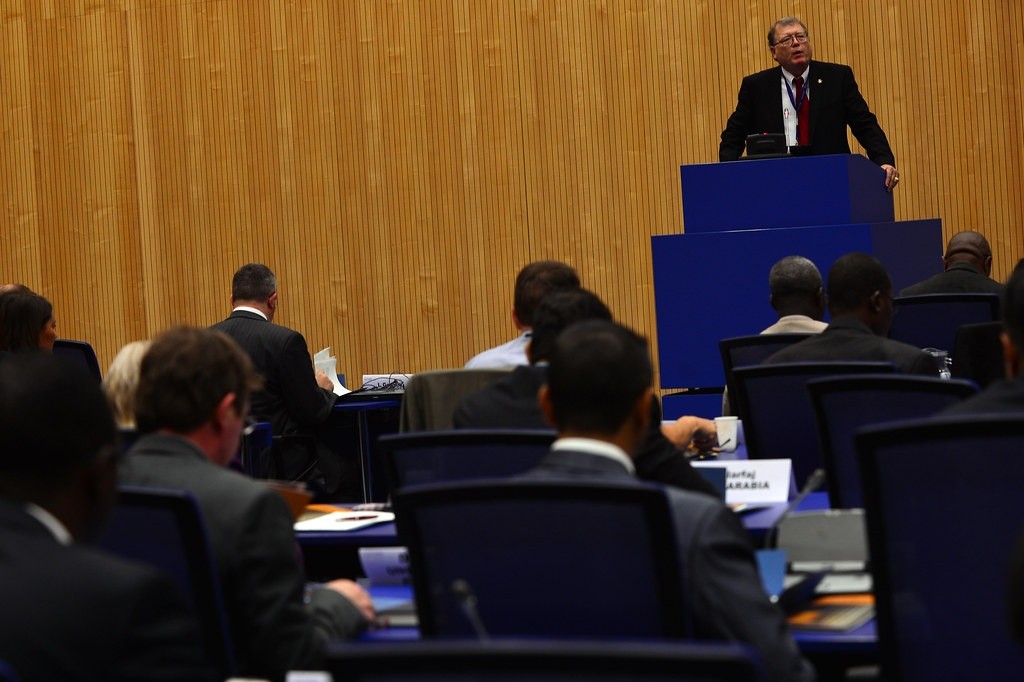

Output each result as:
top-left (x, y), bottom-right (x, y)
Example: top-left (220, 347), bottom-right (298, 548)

top-left (747, 132), bottom-right (786, 156)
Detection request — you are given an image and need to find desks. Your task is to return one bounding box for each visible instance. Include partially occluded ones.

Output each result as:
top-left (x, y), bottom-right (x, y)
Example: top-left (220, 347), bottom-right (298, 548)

top-left (662, 419), bottom-right (753, 467)
top-left (306, 579), bottom-right (420, 645)
top-left (345, 389), bottom-right (403, 401)
top-left (288, 503), bottom-right (398, 579)
top-left (782, 566), bottom-right (881, 682)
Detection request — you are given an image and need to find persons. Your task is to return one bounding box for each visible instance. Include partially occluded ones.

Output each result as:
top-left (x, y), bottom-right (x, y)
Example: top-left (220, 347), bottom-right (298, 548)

top-left (528, 320), bottom-right (816, 682)
top-left (211, 263), bottom-right (339, 434)
top-left (117, 326), bottom-right (373, 682)
top-left (722, 256), bottom-right (829, 416)
top-left (941, 258), bottom-right (1024, 414)
top-left (523, 289), bottom-right (719, 499)
top-left (0, 351), bottom-right (207, 682)
top-left (0, 284), bottom-right (57, 351)
top-left (104, 342), bottom-right (149, 427)
top-left (900, 231), bottom-right (1004, 296)
top-left (465, 260), bottom-right (719, 454)
top-left (763, 253), bottom-right (939, 375)
top-left (719, 17), bottom-right (899, 192)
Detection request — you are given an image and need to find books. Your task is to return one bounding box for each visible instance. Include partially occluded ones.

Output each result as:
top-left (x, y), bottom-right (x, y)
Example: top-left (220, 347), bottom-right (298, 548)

top-left (775, 566), bottom-right (873, 631)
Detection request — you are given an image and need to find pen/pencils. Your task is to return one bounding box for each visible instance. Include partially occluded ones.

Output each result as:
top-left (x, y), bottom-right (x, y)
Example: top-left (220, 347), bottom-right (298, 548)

top-left (342, 515), bottom-right (377, 520)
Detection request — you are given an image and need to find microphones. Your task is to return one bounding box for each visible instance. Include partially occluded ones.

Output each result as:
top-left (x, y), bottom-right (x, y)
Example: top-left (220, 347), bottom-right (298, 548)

top-left (766, 469), bottom-right (826, 549)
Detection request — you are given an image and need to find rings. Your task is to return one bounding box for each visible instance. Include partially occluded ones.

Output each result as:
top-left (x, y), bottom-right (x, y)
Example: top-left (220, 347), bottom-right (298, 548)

top-left (894, 177), bottom-right (899, 183)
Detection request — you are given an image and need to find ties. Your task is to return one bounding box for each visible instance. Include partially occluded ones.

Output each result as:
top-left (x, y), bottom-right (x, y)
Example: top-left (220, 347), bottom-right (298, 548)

top-left (793, 77), bottom-right (811, 146)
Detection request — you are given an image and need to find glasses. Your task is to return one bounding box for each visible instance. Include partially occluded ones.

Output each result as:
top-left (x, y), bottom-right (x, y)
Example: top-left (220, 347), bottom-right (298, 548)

top-left (229, 400), bottom-right (255, 435)
top-left (773, 33), bottom-right (807, 48)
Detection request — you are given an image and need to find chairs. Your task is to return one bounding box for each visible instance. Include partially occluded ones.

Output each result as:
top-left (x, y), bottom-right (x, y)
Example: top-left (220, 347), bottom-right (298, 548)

top-left (0, 293), bottom-right (1024, 682)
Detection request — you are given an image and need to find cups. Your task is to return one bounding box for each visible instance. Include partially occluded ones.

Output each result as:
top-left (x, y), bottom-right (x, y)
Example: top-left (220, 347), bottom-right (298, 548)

top-left (714, 417), bottom-right (738, 452)
